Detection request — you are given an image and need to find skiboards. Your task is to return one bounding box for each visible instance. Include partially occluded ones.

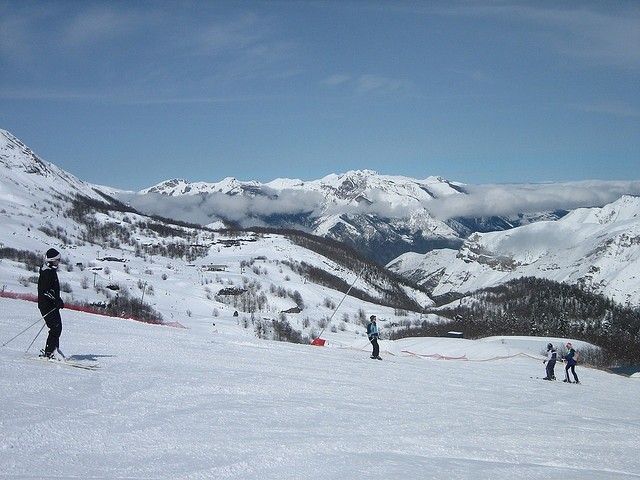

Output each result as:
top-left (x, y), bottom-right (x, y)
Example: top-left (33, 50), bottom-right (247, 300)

top-left (24, 354), bottom-right (102, 371)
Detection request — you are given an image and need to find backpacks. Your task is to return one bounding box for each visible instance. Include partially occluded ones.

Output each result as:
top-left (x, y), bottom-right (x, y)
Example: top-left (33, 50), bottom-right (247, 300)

top-left (572, 351), bottom-right (582, 362)
top-left (366, 323), bottom-right (372, 334)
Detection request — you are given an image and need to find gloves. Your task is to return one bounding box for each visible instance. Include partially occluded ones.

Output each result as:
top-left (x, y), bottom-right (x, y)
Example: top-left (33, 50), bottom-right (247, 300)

top-left (54, 298), bottom-right (64, 310)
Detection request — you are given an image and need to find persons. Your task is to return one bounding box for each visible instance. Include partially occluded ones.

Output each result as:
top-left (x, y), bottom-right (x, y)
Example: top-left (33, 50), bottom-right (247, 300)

top-left (563, 343), bottom-right (579, 383)
top-left (367, 315), bottom-right (380, 358)
top-left (38, 248), bottom-right (64, 359)
top-left (543, 343), bottom-right (557, 380)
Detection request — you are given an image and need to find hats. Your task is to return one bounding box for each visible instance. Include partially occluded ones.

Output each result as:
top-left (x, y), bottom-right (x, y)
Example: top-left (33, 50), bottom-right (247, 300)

top-left (45, 248), bottom-right (61, 262)
top-left (547, 343), bottom-right (552, 348)
top-left (370, 315), bottom-right (376, 321)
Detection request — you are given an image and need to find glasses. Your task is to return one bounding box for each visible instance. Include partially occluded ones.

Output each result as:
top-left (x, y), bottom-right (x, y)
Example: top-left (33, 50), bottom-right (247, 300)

top-left (57, 258), bottom-right (60, 261)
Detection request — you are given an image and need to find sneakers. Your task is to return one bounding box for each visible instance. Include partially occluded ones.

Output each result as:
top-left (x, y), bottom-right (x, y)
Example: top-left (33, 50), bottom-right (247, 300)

top-left (45, 352), bottom-right (56, 359)
top-left (375, 356), bottom-right (382, 360)
top-left (543, 377), bottom-right (551, 380)
top-left (573, 381), bottom-right (580, 384)
top-left (563, 379), bottom-right (571, 383)
top-left (551, 376), bottom-right (556, 380)
